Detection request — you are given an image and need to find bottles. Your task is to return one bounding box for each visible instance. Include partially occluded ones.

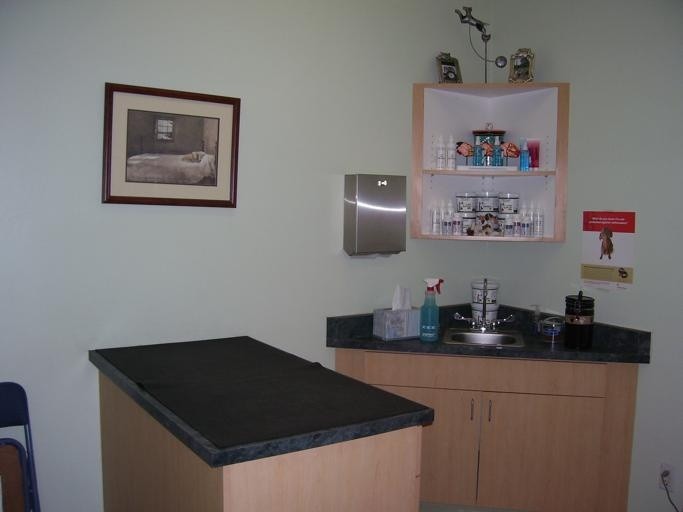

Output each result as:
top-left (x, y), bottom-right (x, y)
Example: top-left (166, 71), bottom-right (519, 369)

top-left (430, 200), bottom-right (546, 237)
top-left (540, 317), bottom-right (564, 343)
top-left (435, 134), bottom-right (530, 172)
top-left (564, 291), bottom-right (595, 326)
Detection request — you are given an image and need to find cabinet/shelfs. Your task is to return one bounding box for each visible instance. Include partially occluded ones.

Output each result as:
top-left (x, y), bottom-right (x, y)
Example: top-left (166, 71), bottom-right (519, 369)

top-left (408, 81), bottom-right (570, 242)
top-left (334, 348), bottom-right (639, 512)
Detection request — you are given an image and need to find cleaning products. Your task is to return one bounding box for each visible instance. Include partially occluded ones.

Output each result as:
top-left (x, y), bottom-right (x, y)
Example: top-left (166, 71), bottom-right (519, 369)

top-left (420, 278), bottom-right (444, 343)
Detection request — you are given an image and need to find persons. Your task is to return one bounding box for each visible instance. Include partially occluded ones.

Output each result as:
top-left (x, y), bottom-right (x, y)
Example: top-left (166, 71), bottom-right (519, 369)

top-left (599, 226), bottom-right (613, 261)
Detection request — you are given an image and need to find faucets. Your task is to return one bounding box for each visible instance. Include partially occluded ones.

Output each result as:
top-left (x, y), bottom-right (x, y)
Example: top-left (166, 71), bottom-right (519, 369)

top-left (481, 278), bottom-right (488, 324)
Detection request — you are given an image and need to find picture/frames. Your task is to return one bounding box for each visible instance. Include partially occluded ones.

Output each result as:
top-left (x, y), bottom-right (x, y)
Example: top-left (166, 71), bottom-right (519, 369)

top-left (509, 47), bottom-right (535, 84)
top-left (436, 53), bottom-right (463, 83)
top-left (102, 82), bottom-right (240, 207)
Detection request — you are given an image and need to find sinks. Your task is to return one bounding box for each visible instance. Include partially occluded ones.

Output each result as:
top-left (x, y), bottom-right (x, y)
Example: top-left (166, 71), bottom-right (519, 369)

top-left (441, 327), bottom-right (526, 349)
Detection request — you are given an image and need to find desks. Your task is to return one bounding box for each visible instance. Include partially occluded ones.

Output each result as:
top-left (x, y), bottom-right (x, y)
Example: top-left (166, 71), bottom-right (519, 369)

top-left (88, 336), bottom-right (436, 512)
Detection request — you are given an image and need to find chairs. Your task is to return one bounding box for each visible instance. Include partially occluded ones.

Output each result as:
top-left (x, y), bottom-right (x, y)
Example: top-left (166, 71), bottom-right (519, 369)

top-left (0, 381), bottom-right (41, 512)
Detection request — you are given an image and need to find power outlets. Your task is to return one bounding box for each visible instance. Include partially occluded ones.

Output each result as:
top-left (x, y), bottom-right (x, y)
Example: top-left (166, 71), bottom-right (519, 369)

top-left (658, 463), bottom-right (673, 492)
